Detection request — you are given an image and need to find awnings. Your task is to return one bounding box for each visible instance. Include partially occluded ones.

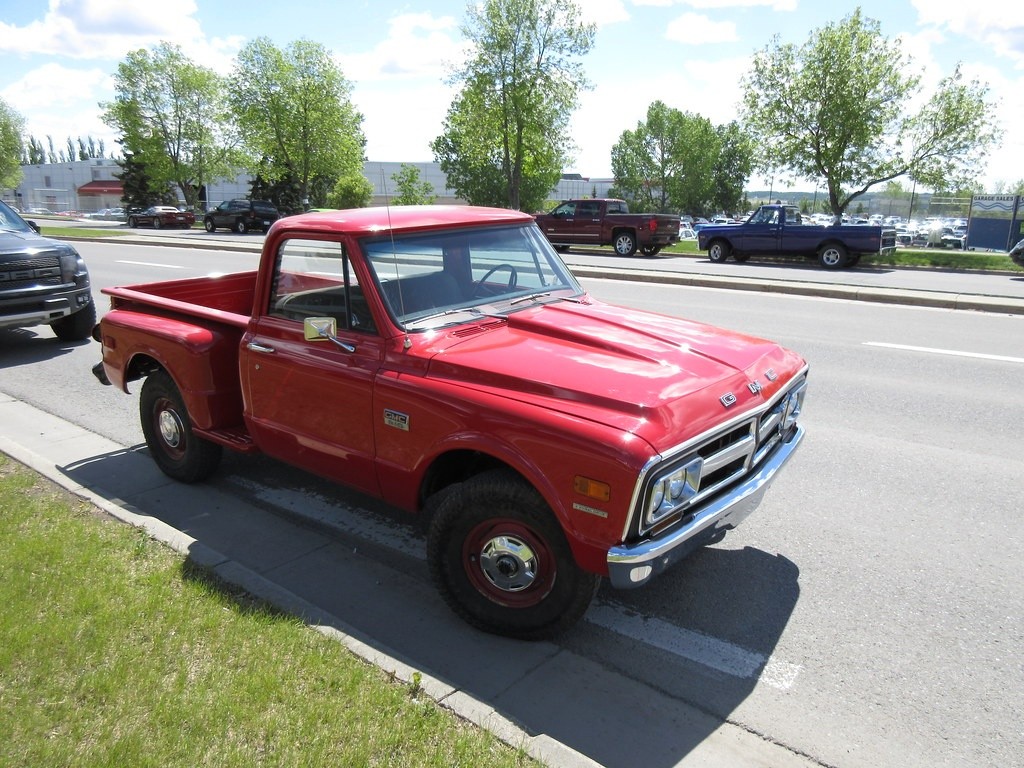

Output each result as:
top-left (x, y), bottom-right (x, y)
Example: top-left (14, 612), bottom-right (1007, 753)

top-left (79, 181), bottom-right (125, 194)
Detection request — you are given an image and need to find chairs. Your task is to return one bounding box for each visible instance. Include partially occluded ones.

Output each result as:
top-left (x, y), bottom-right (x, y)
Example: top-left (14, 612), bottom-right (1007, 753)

top-left (582, 206), bottom-right (592, 216)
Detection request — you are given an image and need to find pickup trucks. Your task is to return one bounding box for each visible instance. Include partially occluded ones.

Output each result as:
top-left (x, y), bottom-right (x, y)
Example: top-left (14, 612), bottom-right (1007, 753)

top-left (91, 202), bottom-right (811, 643)
top-left (698, 204), bottom-right (898, 270)
top-left (528, 197), bottom-right (683, 258)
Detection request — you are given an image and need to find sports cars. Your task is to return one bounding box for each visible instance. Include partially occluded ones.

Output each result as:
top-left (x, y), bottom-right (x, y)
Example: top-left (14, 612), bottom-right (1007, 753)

top-left (128, 205), bottom-right (195, 228)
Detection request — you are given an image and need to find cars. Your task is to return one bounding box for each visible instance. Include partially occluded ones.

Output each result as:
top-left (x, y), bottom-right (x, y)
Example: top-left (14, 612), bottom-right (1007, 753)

top-left (306, 209), bottom-right (337, 213)
top-left (677, 212), bottom-right (969, 249)
top-left (9, 205), bottom-right (138, 221)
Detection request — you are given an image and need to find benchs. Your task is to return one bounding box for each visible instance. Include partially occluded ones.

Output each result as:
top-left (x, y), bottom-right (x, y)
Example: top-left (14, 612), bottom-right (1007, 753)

top-left (273, 272), bottom-right (462, 330)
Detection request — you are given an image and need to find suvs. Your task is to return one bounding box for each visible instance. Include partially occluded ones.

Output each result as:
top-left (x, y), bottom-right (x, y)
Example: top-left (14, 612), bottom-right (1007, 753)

top-left (203, 198), bottom-right (280, 235)
top-left (0, 200), bottom-right (99, 341)
